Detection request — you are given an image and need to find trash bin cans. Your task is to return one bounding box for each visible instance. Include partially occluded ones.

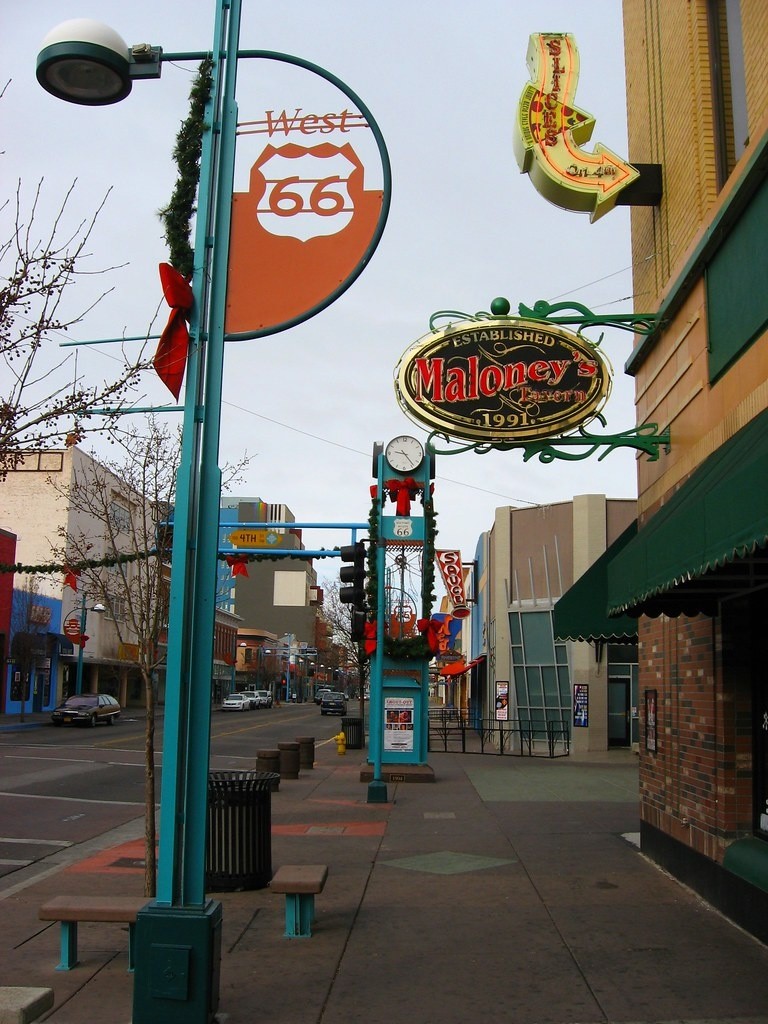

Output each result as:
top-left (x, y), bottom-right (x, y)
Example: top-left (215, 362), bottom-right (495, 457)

top-left (205, 770), bottom-right (281, 890)
top-left (341, 717), bottom-right (363, 749)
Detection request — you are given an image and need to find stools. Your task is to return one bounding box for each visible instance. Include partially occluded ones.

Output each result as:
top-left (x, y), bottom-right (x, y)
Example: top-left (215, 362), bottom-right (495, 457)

top-left (0, 983), bottom-right (55, 1024)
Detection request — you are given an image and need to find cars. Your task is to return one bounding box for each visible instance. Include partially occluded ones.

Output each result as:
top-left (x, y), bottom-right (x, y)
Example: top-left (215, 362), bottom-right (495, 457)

top-left (51, 692), bottom-right (120, 726)
top-left (315, 689), bottom-right (331, 704)
top-left (342, 693), bottom-right (349, 700)
top-left (222, 694), bottom-right (251, 711)
top-left (358, 694), bottom-right (370, 700)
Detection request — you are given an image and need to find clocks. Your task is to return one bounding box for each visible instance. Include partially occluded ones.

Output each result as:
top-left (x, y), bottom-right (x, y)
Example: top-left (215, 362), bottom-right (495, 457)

top-left (386, 436), bottom-right (425, 474)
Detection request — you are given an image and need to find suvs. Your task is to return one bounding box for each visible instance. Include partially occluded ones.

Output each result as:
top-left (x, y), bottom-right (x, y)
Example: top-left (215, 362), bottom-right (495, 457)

top-left (242, 690), bottom-right (261, 709)
top-left (321, 692), bottom-right (348, 716)
top-left (256, 690), bottom-right (272, 708)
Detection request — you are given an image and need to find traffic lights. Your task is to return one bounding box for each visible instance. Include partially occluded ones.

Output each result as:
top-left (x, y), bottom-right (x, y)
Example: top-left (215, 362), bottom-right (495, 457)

top-left (283, 678), bottom-right (286, 684)
top-left (336, 541), bottom-right (366, 603)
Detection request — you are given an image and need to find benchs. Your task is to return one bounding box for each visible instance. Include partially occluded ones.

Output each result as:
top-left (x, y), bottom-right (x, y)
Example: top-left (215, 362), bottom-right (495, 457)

top-left (38, 896), bottom-right (154, 972)
top-left (269, 865), bottom-right (328, 939)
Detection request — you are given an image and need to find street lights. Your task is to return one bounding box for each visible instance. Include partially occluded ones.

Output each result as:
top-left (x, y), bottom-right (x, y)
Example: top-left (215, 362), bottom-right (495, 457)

top-left (62, 602), bottom-right (107, 695)
top-left (224, 642), bottom-right (246, 692)
top-left (34, 19), bottom-right (390, 1023)
top-left (250, 650), bottom-right (272, 689)
top-left (284, 633), bottom-right (290, 701)
top-left (281, 656), bottom-right (344, 703)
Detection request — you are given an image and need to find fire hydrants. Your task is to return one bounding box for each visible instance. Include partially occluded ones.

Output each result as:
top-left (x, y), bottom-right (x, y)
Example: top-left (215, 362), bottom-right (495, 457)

top-left (291, 693), bottom-right (297, 703)
top-left (334, 734), bottom-right (347, 756)
